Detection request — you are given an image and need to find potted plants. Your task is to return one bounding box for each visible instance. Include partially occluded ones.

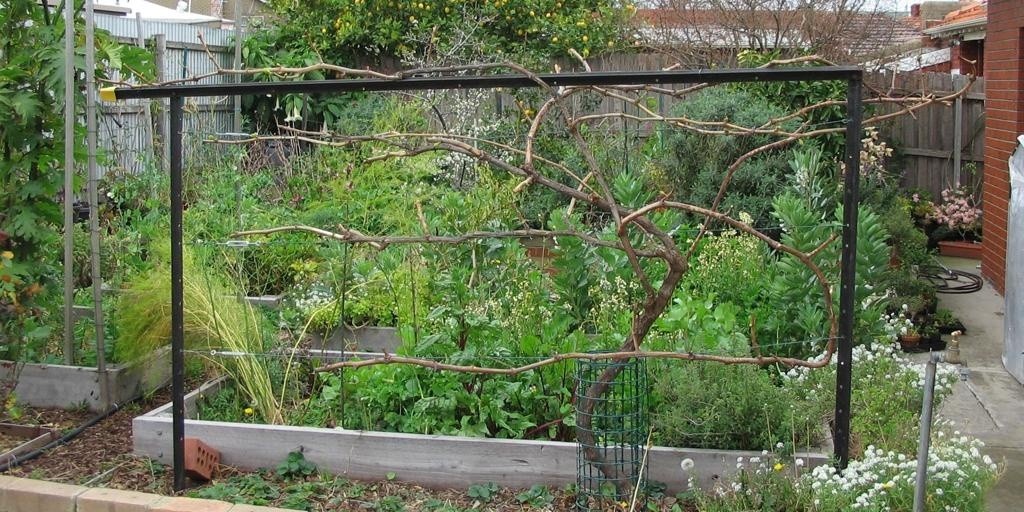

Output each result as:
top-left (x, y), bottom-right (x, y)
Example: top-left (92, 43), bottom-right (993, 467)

top-left (898, 308), bottom-right (968, 353)
